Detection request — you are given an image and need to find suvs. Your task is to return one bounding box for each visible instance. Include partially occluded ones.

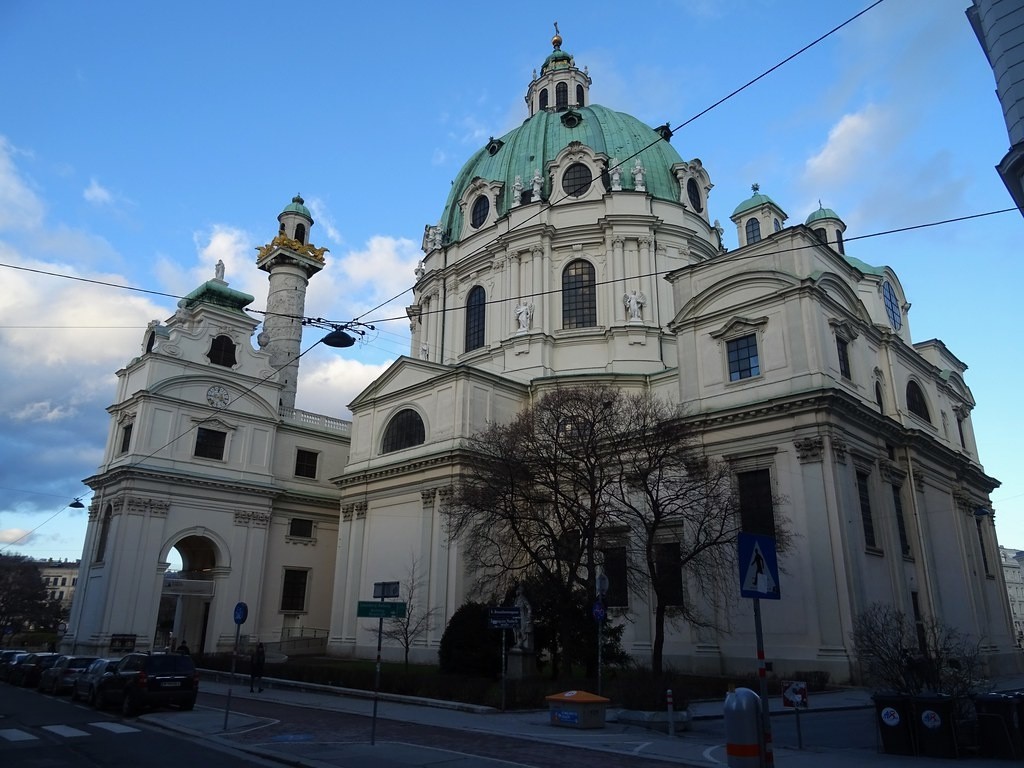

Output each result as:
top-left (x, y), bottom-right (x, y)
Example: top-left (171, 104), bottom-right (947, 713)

top-left (100, 653), bottom-right (199, 719)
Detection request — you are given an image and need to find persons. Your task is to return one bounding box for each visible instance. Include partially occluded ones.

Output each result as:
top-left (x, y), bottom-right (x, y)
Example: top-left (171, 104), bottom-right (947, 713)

top-left (414, 258), bottom-right (424, 280)
top-left (177, 640), bottom-right (190, 655)
top-left (509, 174), bottom-right (523, 207)
top-left (529, 169), bottom-right (544, 202)
top-left (248, 642), bottom-right (265, 693)
top-left (606, 156), bottom-right (624, 191)
top-left (515, 301), bottom-right (533, 332)
top-left (48, 642), bottom-right (56, 652)
top-left (426, 222), bottom-right (442, 253)
top-left (214, 259), bottom-right (226, 280)
top-left (418, 339), bottom-right (429, 362)
top-left (630, 156), bottom-right (647, 192)
top-left (621, 288), bottom-right (646, 322)
top-left (917, 658), bottom-right (932, 691)
top-left (713, 218), bottom-right (725, 249)
top-left (513, 585), bottom-right (535, 653)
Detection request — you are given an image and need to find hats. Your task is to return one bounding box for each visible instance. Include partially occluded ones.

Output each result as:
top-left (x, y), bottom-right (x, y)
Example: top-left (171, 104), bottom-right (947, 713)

top-left (181, 640), bottom-right (186, 644)
top-left (257, 643), bottom-right (263, 649)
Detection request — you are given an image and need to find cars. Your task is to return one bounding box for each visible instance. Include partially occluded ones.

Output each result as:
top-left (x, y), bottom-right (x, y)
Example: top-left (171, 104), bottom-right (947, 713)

top-left (72, 656), bottom-right (124, 707)
top-left (0, 649), bottom-right (99, 697)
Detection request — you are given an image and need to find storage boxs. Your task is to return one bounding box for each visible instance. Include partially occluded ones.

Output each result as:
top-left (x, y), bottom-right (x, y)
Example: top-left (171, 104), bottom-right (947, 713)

top-left (545, 691), bottom-right (609, 729)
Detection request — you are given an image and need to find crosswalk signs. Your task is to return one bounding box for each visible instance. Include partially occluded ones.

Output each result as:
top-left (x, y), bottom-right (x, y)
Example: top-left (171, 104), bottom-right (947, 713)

top-left (734, 534), bottom-right (783, 601)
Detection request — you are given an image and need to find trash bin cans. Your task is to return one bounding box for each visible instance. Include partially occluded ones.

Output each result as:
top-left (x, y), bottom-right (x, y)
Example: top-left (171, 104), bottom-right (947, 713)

top-left (724, 687), bottom-right (766, 768)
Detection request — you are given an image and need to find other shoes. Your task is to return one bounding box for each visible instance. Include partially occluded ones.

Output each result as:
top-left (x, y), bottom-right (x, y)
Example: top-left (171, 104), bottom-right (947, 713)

top-left (250, 687), bottom-right (254, 693)
top-left (258, 687), bottom-right (264, 693)
